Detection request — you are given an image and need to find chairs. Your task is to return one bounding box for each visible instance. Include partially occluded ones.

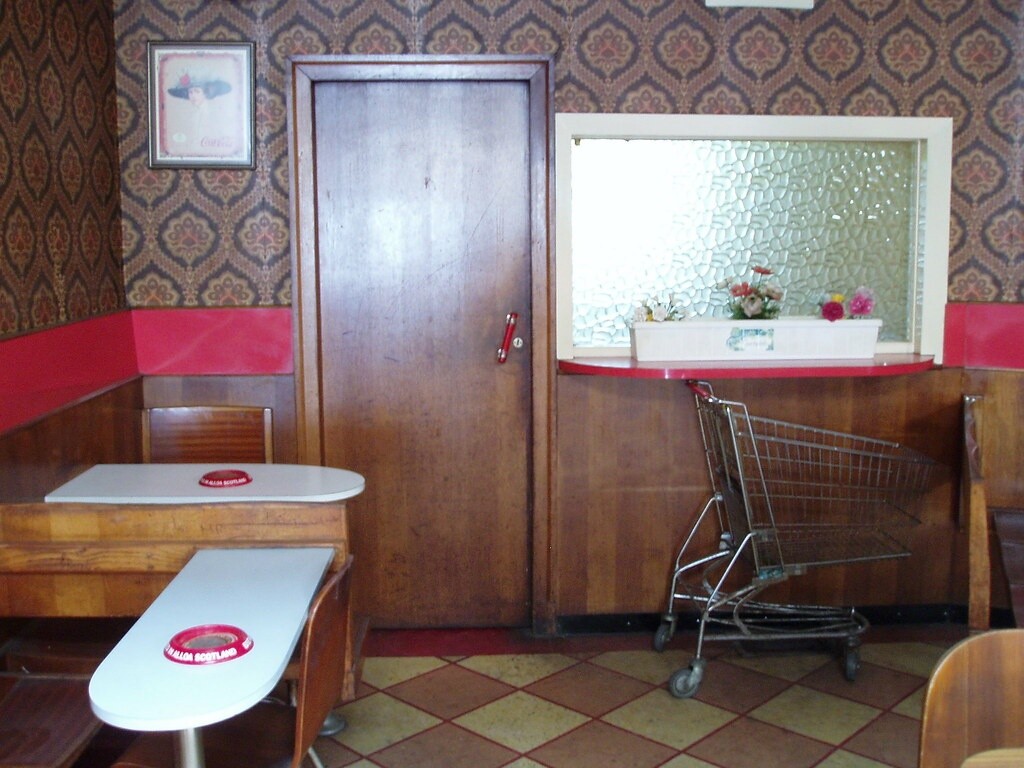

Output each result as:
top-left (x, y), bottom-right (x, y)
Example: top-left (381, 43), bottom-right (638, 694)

top-left (142, 404), bottom-right (272, 463)
top-left (917, 628), bottom-right (1023, 768)
top-left (959, 392), bottom-right (1024, 639)
top-left (111, 553), bottom-right (354, 768)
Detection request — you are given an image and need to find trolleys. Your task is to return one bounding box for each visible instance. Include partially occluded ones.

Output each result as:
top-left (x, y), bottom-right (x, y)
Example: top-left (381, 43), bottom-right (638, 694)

top-left (653, 379), bottom-right (940, 702)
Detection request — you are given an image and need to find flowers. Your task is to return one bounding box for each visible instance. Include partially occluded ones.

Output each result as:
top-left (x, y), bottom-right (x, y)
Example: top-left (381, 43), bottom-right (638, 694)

top-left (632, 292), bottom-right (685, 321)
top-left (818, 286), bottom-right (875, 322)
top-left (715, 265), bottom-right (784, 320)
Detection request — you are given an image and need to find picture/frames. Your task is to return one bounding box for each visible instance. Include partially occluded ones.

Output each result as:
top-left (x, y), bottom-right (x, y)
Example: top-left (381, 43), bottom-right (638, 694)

top-left (147, 40), bottom-right (257, 170)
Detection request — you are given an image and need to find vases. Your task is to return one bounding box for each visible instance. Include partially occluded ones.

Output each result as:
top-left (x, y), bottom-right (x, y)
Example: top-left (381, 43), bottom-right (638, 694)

top-left (626, 315), bottom-right (883, 362)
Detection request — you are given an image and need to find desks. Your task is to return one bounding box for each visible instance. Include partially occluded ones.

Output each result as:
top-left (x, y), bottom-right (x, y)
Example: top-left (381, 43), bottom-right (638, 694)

top-left (43, 463), bottom-right (367, 503)
top-left (88, 547), bottom-right (337, 768)
